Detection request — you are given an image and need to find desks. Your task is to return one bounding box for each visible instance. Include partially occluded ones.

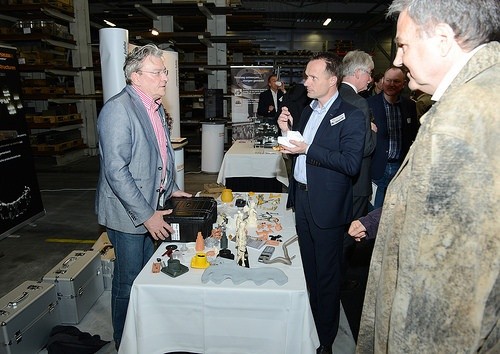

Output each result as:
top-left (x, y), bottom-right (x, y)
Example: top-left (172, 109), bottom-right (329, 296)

top-left (217, 140), bottom-right (288, 192)
top-left (116, 191), bottom-right (321, 354)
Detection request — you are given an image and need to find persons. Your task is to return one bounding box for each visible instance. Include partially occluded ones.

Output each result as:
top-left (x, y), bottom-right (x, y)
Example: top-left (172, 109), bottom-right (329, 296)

top-left (258, 50), bottom-right (434, 354)
top-left (356, 1), bottom-right (499, 353)
top-left (95, 44), bottom-right (191, 352)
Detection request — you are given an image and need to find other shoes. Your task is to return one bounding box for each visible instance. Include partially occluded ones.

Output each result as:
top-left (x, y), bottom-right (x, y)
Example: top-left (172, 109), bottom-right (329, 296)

top-left (316, 345), bottom-right (332, 354)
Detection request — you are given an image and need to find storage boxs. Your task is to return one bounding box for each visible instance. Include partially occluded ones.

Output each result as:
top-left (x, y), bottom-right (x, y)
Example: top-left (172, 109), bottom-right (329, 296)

top-left (161, 197), bottom-right (217, 242)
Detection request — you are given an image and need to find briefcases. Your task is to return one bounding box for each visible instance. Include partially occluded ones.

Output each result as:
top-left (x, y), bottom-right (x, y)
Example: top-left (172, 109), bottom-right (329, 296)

top-left (160, 196), bottom-right (218, 242)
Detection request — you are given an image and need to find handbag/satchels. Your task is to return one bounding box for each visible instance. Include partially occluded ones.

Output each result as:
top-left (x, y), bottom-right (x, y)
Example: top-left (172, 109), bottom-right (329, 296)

top-left (46, 325), bottom-right (112, 354)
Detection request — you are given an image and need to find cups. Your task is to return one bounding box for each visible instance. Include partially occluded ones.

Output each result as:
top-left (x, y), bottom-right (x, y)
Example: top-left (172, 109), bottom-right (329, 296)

top-left (274, 81), bottom-right (282, 86)
top-left (287, 130), bottom-right (301, 147)
top-left (221, 189), bottom-right (233, 203)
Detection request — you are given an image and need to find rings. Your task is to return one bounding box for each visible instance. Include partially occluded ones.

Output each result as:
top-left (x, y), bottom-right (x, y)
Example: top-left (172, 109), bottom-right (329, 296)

top-left (157, 232), bottom-right (161, 235)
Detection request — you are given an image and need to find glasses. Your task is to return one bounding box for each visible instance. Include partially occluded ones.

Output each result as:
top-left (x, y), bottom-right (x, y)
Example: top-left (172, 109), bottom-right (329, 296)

top-left (359, 68), bottom-right (373, 77)
top-left (137, 68), bottom-right (169, 78)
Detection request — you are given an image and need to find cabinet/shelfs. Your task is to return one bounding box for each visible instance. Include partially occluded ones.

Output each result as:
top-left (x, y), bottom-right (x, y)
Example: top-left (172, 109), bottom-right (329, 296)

top-left (249, 55), bottom-right (306, 77)
top-left (0, 3), bottom-right (102, 168)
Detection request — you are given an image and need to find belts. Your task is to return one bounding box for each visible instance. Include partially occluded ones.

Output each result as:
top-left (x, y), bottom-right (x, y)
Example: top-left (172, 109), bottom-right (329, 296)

top-left (291, 177), bottom-right (308, 192)
